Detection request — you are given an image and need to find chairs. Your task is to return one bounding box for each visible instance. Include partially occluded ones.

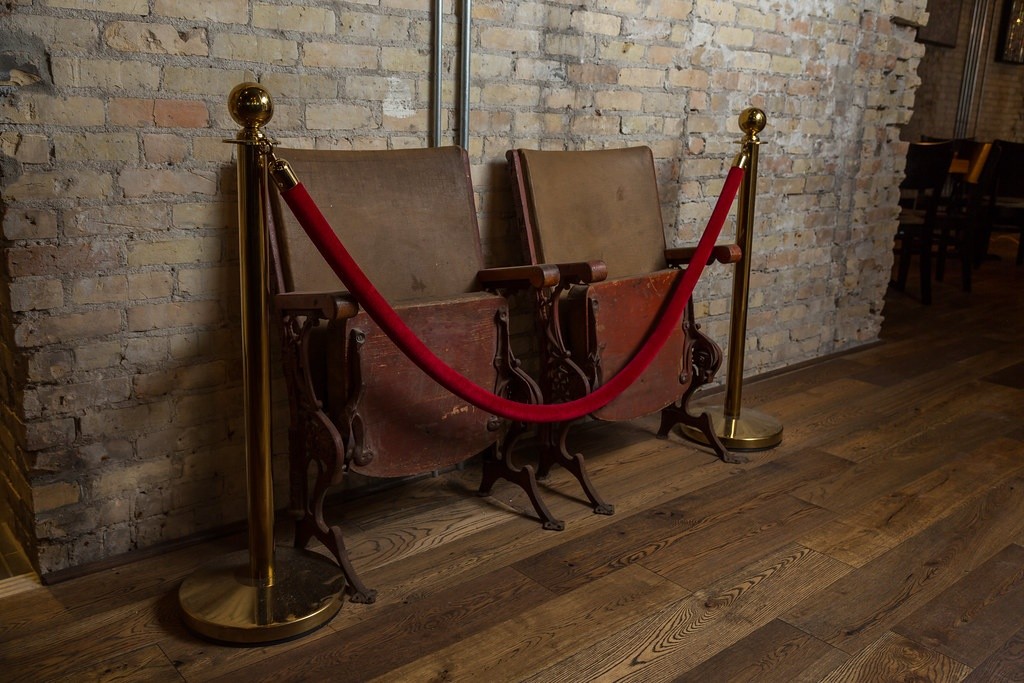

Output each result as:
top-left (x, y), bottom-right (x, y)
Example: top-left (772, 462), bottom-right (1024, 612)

top-left (506, 144), bottom-right (750, 517)
top-left (894, 138), bottom-right (954, 306)
top-left (265, 144), bottom-right (568, 604)
top-left (935, 143), bottom-right (1003, 294)
